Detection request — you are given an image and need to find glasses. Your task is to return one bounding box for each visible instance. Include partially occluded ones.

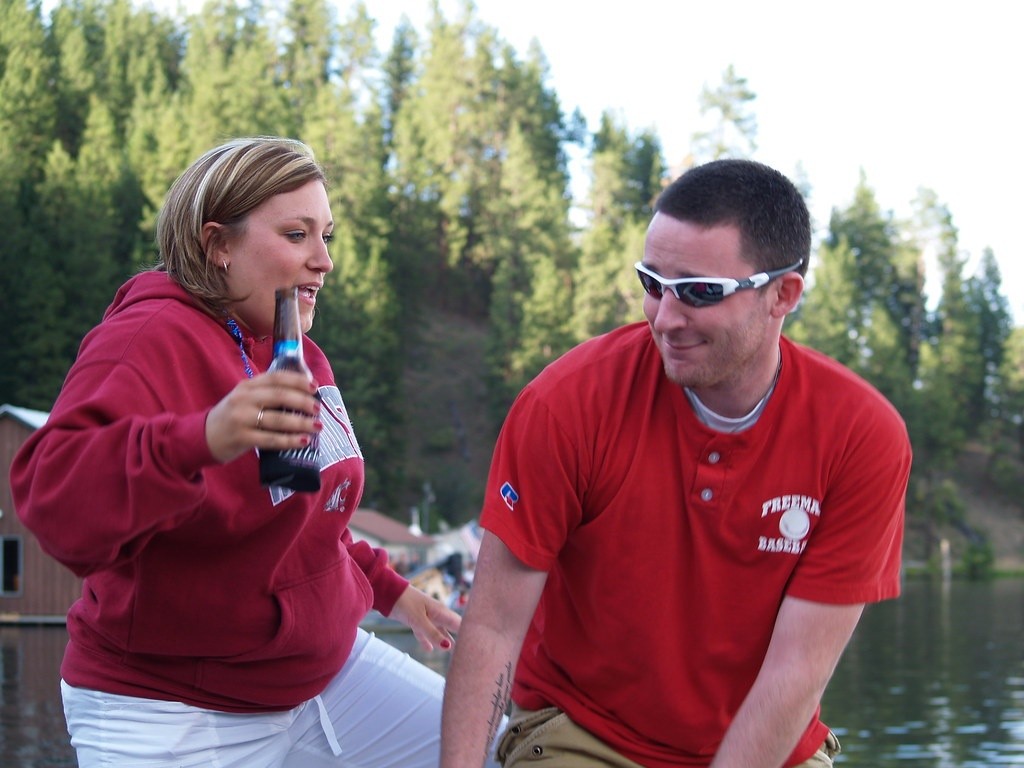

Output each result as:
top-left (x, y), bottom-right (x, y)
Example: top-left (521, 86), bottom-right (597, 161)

top-left (633, 257), bottom-right (805, 308)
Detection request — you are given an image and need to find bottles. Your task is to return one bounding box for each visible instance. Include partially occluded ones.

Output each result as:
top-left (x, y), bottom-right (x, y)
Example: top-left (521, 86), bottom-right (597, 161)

top-left (260, 288), bottom-right (321, 494)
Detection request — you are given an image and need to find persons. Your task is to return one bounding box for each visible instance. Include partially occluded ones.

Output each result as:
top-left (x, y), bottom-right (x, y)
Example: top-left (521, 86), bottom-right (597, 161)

top-left (7, 136), bottom-right (511, 768)
top-left (439, 159), bottom-right (912, 768)
top-left (388, 549), bottom-right (420, 575)
top-left (460, 561), bottom-right (475, 583)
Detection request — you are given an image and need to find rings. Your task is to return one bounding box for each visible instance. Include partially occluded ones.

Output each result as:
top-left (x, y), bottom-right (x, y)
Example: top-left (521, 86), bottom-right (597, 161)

top-left (256, 408), bottom-right (264, 428)
